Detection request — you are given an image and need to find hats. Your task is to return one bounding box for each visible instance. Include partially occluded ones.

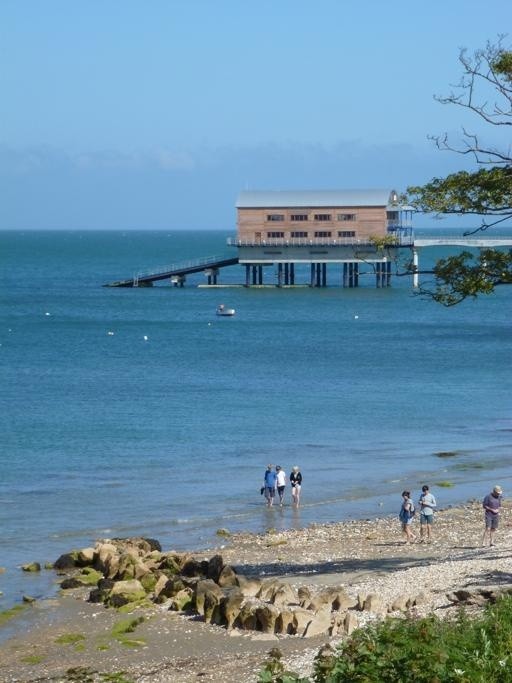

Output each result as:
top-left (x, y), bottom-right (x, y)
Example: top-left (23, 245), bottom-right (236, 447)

top-left (495, 486), bottom-right (502, 494)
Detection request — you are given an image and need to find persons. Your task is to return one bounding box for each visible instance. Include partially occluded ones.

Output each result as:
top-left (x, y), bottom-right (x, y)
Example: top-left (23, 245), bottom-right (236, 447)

top-left (289, 466), bottom-right (302, 507)
top-left (273, 466), bottom-right (288, 506)
top-left (399, 490), bottom-right (417, 545)
top-left (418, 485), bottom-right (437, 540)
top-left (260, 464), bottom-right (276, 507)
top-left (481, 484), bottom-right (503, 547)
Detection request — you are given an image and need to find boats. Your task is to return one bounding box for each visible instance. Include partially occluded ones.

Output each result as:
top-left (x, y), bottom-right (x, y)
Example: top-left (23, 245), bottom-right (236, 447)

top-left (215, 304), bottom-right (235, 315)
